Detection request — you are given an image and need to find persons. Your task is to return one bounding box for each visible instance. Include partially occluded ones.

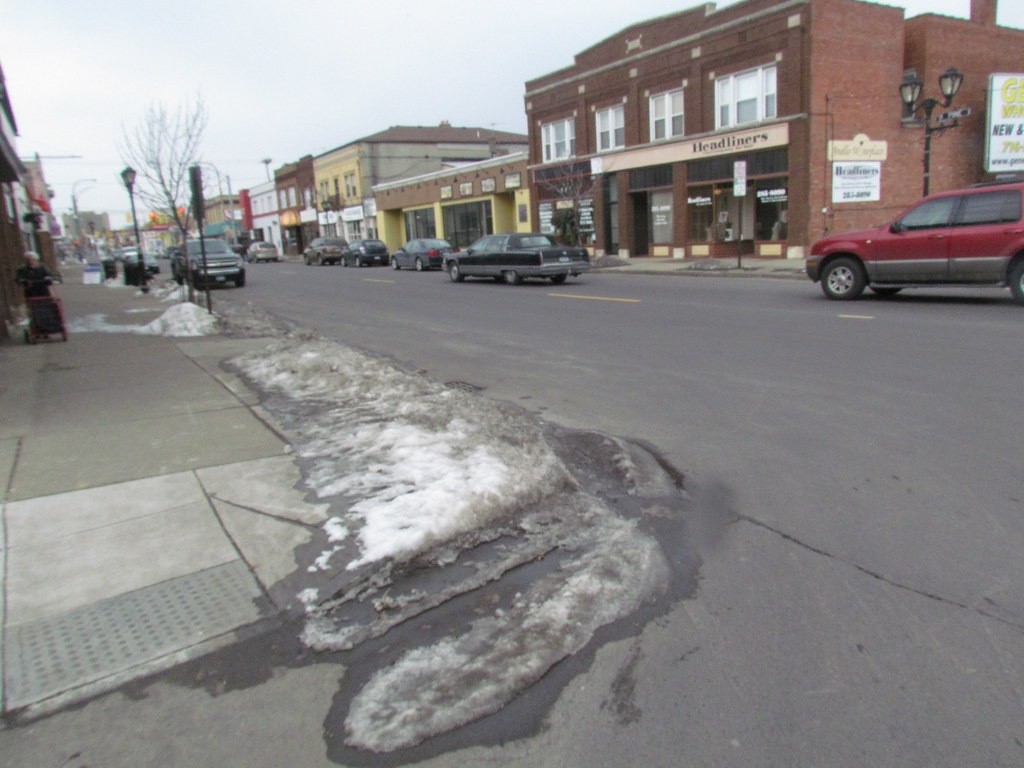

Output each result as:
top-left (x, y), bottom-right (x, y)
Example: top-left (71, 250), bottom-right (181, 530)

top-left (14, 251), bottom-right (54, 341)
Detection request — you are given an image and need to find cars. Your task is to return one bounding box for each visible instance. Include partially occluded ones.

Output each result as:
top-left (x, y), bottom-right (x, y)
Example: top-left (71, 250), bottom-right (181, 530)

top-left (128, 254), bottom-right (159, 281)
top-left (246, 241), bottom-right (280, 264)
top-left (172, 237), bottom-right (245, 289)
top-left (443, 231), bottom-right (590, 284)
top-left (302, 236), bottom-right (348, 265)
top-left (121, 246), bottom-right (139, 261)
top-left (339, 239), bottom-right (389, 268)
top-left (391, 238), bottom-right (458, 272)
top-left (801, 180), bottom-right (1023, 302)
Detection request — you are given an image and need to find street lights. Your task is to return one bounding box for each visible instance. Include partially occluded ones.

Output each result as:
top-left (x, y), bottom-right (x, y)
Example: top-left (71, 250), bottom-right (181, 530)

top-left (899, 65), bottom-right (963, 196)
top-left (120, 166), bottom-right (148, 288)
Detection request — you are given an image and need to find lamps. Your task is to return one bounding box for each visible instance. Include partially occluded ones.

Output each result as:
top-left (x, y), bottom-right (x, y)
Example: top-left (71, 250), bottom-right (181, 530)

top-left (474, 165), bottom-right (489, 178)
top-left (434, 175), bottom-right (447, 185)
top-left (500, 159), bottom-right (514, 174)
top-left (417, 178), bottom-right (429, 189)
top-left (508, 186), bottom-right (523, 197)
top-left (401, 182), bottom-right (413, 192)
top-left (453, 171), bottom-right (467, 182)
top-left (386, 184), bottom-right (397, 196)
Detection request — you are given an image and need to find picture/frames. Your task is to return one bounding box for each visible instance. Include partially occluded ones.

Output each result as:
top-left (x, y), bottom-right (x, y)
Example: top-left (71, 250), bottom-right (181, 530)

top-left (440, 184), bottom-right (453, 199)
top-left (481, 176), bottom-right (497, 194)
top-left (504, 172), bottom-right (521, 189)
top-left (459, 181), bottom-right (473, 197)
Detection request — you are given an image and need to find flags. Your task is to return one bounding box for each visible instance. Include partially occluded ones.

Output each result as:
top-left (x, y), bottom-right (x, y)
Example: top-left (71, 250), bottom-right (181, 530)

top-left (33, 196), bottom-right (50, 212)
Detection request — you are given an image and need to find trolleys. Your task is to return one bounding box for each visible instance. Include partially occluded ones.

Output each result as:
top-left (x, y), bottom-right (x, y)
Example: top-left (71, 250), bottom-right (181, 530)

top-left (20, 278), bottom-right (70, 343)
